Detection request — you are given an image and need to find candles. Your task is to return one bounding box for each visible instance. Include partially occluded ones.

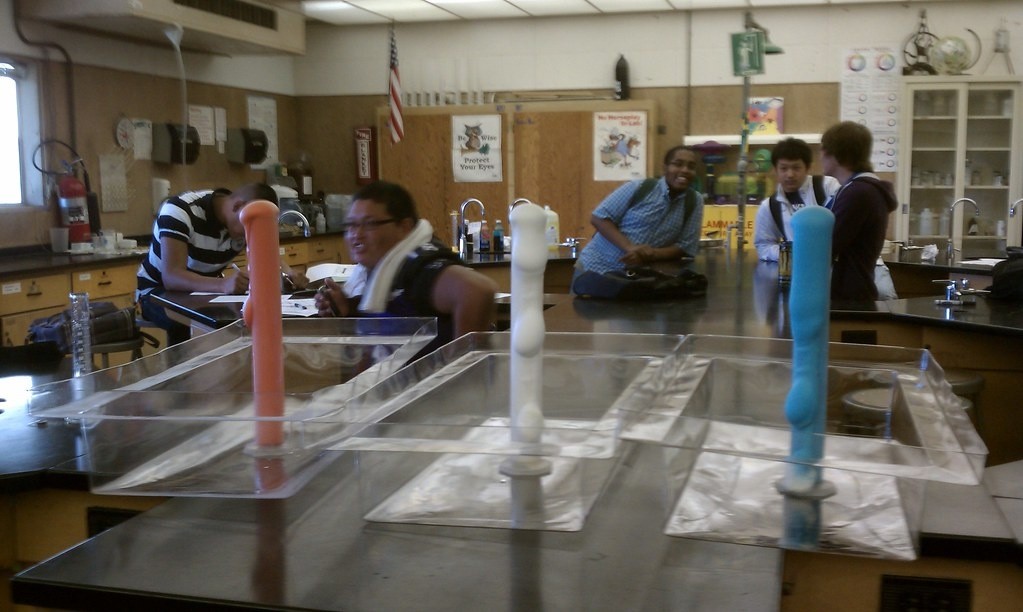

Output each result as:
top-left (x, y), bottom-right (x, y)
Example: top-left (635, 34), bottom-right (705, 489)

top-left (240, 202), bottom-right (287, 445)
top-left (787, 202), bottom-right (836, 487)
top-left (511, 203), bottom-right (548, 456)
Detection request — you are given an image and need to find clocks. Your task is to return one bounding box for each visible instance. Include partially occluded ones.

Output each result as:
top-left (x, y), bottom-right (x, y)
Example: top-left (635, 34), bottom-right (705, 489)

top-left (113, 112), bottom-right (136, 149)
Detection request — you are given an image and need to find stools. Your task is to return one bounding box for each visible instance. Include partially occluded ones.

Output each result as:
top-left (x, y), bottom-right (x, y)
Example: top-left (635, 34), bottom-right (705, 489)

top-left (837, 368), bottom-right (983, 437)
top-left (86, 337), bottom-right (147, 382)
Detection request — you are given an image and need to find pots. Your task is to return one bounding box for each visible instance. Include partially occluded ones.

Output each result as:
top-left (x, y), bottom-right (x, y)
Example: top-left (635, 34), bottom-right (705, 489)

top-left (889, 240), bottom-right (925, 262)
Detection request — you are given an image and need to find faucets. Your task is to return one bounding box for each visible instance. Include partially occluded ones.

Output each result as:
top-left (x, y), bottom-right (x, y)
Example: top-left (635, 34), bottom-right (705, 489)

top-left (459, 197), bottom-right (485, 254)
top-left (943, 197), bottom-right (980, 258)
top-left (932, 279), bottom-right (961, 301)
top-left (555, 237), bottom-right (587, 250)
top-left (277, 210), bottom-right (312, 299)
top-left (1009, 199), bottom-right (1023, 218)
top-left (508, 198), bottom-right (531, 230)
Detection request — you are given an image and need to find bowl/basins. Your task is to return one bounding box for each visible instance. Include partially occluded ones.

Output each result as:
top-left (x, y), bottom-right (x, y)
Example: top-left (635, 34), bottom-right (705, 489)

top-left (119, 240), bottom-right (137, 248)
top-left (71, 243), bottom-right (92, 250)
top-left (699, 239), bottom-right (724, 247)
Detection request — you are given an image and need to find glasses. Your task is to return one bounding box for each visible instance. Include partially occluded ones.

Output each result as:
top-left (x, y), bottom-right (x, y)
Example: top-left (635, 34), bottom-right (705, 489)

top-left (340, 218), bottom-right (394, 235)
top-left (666, 159), bottom-right (698, 170)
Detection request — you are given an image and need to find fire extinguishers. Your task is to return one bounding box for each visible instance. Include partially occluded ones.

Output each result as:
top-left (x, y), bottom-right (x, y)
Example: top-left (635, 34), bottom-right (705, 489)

top-left (32, 139), bottom-right (101, 249)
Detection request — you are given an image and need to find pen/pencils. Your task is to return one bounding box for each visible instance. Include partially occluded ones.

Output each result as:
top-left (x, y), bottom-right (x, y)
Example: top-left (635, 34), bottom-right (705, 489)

top-left (231, 263), bottom-right (241, 272)
top-left (934, 298), bottom-right (977, 306)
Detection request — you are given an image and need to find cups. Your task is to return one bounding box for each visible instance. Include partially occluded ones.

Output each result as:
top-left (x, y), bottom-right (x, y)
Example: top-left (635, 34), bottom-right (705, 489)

top-left (83, 233), bottom-right (96, 249)
top-left (468, 221), bottom-right (481, 252)
top-left (778, 241), bottom-right (792, 291)
top-left (50, 228), bottom-right (70, 252)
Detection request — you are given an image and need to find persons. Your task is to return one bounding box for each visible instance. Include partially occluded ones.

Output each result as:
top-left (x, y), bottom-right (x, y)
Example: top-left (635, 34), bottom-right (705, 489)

top-left (569, 146), bottom-right (704, 295)
top-left (135, 183), bottom-right (310, 348)
top-left (754, 138), bottom-right (898, 299)
top-left (820, 121), bottom-right (898, 343)
top-left (315, 181), bottom-right (496, 384)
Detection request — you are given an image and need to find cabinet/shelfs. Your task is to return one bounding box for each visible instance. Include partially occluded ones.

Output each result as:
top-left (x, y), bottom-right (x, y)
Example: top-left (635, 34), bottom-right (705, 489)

top-left (1, 265), bottom-right (147, 379)
top-left (826, 316), bottom-right (1022, 464)
top-left (223, 232), bottom-right (357, 278)
top-left (896, 75), bottom-right (1023, 248)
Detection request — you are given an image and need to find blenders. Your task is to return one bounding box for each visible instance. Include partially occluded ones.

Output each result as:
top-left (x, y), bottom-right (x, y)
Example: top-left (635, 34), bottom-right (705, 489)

top-left (267, 155), bottom-right (302, 232)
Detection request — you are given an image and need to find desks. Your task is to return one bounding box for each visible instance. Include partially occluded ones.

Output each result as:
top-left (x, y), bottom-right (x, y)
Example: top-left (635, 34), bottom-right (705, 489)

top-left (456, 246), bottom-right (583, 298)
top-left (149, 275), bottom-right (347, 392)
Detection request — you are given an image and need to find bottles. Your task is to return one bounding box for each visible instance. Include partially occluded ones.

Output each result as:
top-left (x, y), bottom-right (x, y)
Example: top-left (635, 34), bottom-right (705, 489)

top-left (911, 164), bottom-right (953, 186)
top-left (969, 217), bottom-right (977, 235)
top-left (755, 153), bottom-right (765, 194)
top-left (493, 220), bottom-right (504, 251)
top-left (913, 91), bottom-right (1013, 115)
top-left (317, 213), bottom-right (326, 233)
top-left (98, 225), bottom-right (104, 241)
top-left (289, 153), bottom-right (316, 204)
top-left (745, 160), bottom-right (757, 194)
top-left (543, 205), bottom-right (559, 250)
top-left (706, 163), bottom-right (715, 204)
top-left (104, 233), bottom-right (113, 250)
top-left (615, 53), bottom-right (629, 100)
top-left (965, 159), bottom-right (981, 186)
top-left (997, 220), bottom-right (1005, 235)
top-left (464, 220), bottom-right (469, 236)
top-left (939, 208), bottom-right (950, 236)
top-left (992, 170), bottom-right (1004, 186)
top-left (117, 230), bottom-right (123, 243)
top-left (318, 191), bottom-right (323, 205)
top-left (480, 220), bottom-right (490, 251)
top-left (921, 208), bottom-right (933, 235)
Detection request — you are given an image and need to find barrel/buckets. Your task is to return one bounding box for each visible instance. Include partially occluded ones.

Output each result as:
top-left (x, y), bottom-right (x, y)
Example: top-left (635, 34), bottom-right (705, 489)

top-left (869, 365), bottom-right (985, 429)
top-left (840, 387), bottom-right (974, 440)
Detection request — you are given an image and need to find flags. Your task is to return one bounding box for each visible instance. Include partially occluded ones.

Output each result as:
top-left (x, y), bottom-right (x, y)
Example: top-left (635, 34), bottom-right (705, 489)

top-left (387, 19), bottom-right (405, 145)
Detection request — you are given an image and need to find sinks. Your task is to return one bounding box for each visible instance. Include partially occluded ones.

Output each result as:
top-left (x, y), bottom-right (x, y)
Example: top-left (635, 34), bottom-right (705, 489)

top-left (964, 256), bottom-right (1008, 263)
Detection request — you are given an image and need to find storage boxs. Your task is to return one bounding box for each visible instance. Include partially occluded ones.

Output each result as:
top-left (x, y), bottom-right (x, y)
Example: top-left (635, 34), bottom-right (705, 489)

top-left (27, 308), bottom-right (987, 563)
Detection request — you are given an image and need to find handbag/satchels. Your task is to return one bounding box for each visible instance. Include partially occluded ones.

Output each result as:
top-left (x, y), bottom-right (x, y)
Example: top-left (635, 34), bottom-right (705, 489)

top-left (572, 256), bottom-right (709, 300)
top-left (22, 299), bottom-right (159, 353)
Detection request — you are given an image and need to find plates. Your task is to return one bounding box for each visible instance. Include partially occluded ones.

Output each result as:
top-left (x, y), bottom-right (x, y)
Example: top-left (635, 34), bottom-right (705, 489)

top-left (64, 250), bottom-right (97, 254)
